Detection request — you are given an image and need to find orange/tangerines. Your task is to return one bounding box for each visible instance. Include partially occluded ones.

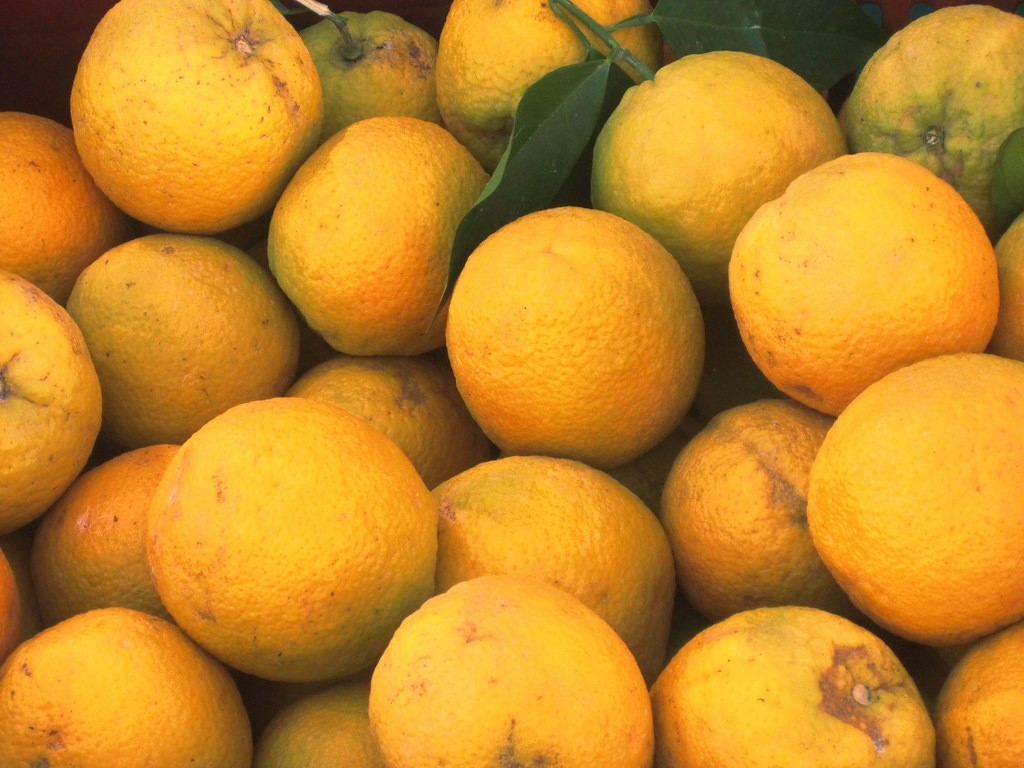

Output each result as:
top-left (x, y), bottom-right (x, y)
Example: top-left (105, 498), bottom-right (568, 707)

top-left (0, 0), bottom-right (1024, 768)
